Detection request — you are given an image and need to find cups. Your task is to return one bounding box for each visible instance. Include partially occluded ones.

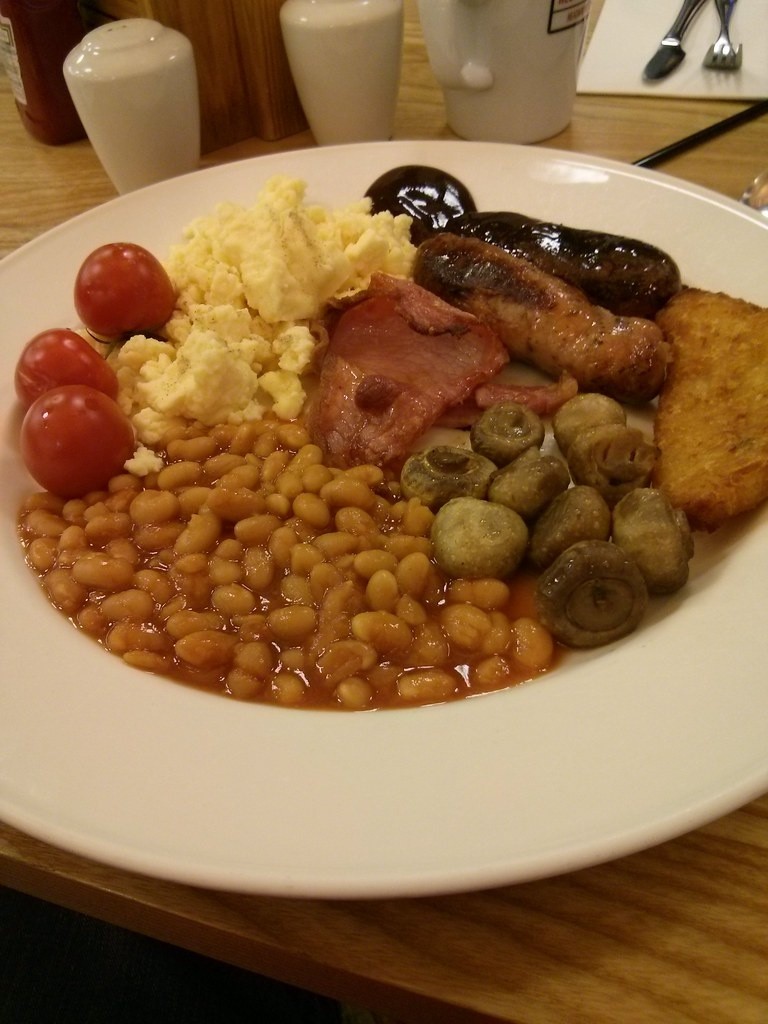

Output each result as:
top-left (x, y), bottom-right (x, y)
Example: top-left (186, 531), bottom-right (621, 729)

top-left (414, 3), bottom-right (578, 145)
top-left (278, 3), bottom-right (403, 143)
top-left (63, 20), bottom-right (205, 196)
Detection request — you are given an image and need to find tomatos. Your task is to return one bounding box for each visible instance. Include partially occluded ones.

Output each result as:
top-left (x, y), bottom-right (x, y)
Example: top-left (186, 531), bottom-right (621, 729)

top-left (13, 239), bottom-right (174, 499)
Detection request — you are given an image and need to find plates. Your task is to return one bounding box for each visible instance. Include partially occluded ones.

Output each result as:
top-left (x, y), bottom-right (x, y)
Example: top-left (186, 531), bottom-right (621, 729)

top-left (0, 134), bottom-right (767, 897)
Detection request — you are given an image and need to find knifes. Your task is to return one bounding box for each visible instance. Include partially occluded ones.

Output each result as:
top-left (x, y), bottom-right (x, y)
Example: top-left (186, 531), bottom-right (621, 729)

top-left (644, 0), bottom-right (705, 80)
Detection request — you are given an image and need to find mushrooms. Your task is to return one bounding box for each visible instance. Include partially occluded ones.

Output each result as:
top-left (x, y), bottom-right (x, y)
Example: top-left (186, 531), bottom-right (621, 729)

top-left (400, 387), bottom-right (690, 653)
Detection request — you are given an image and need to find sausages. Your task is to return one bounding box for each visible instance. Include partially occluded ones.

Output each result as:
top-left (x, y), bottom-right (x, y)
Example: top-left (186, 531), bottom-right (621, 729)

top-left (415, 210), bottom-right (681, 405)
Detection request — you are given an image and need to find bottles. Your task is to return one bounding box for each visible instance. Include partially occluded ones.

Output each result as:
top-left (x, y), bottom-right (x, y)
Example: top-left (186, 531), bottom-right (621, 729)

top-left (0, 4), bottom-right (99, 147)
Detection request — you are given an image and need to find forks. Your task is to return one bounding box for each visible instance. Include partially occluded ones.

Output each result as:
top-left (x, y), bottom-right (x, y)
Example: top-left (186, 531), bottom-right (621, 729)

top-left (703, 0), bottom-right (745, 72)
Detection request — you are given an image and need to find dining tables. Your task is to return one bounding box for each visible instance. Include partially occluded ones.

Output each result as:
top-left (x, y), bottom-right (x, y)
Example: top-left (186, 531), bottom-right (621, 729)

top-left (0, 2), bottom-right (764, 1022)
